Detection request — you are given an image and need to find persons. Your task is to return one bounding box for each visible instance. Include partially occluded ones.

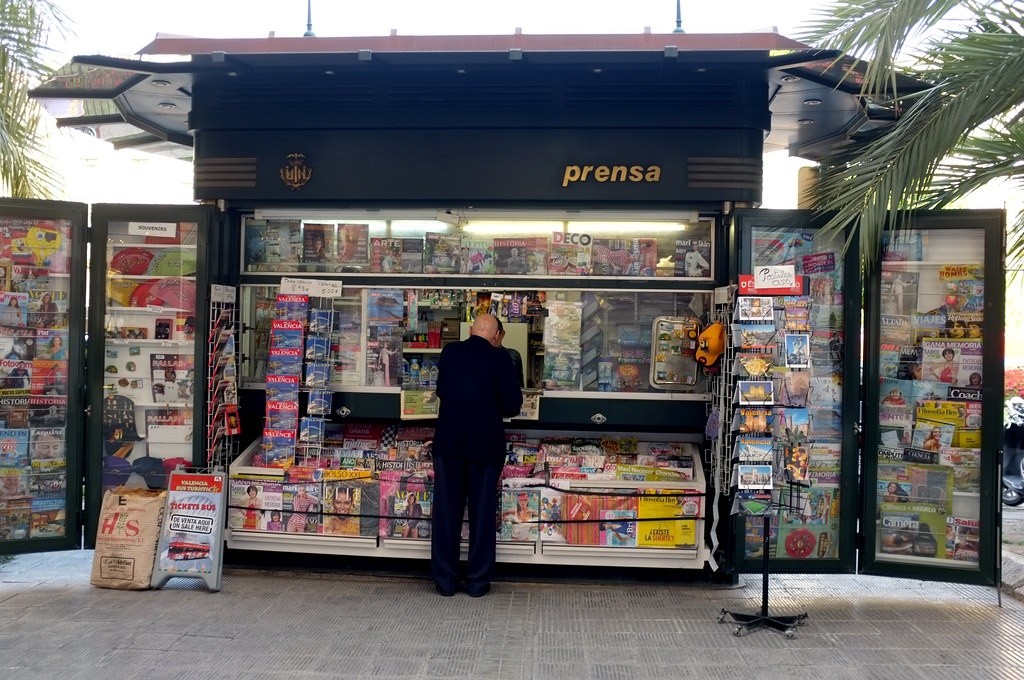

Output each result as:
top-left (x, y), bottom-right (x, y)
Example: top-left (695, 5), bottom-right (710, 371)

top-left (30, 429), bottom-right (63, 463)
top-left (593, 468), bottom-right (603, 480)
top-left (965, 373), bottom-right (981, 388)
top-left (11, 361), bottom-right (30, 388)
top-left (237, 486), bottom-right (263, 529)
top-left (883, 483), bottom-right (909, 503)
top-left (3, 297), bottom-right (22, 325)
top-left (502, 494), bottom-right (537, 540)
top-left (493, 316), bottom-right (525, 387)
top-left (600, 516), bottom-right (636, 542)
top-left (494, 248), bottom-right (524, 273)
top-left (267, 509), bottom-right (283, 531)
top-left (35, 293), bottom-right (58, 326)
top-left (49, 335), bottom-right (68, 360)
top-left (930, 348), bottom-right (959, 385)
top-left (923, 427), bottom-right (943, 452)
top-left (967, 322), bottom-right (982, 337)
top-left (325, 487), bottom-right (359, 535)
top-left (402, 494), bottom-right (422, 538)
top-left (287, 485), bottom-right (318, 532)
top-left (432, 314), bottom-right (523, 596)
top-left (946, 320), bottom-right (967, 337)
top-left (1, 472), bottom-right (25, 499)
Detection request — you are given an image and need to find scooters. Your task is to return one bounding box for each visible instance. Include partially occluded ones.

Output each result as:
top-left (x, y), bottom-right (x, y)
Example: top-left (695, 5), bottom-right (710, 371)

top-left (1002, 396), bottom-right (1024, 507)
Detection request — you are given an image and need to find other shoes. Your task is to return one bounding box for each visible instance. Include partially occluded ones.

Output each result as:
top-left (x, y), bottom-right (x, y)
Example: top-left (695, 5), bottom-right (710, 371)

top-left (469, 583), bottom-right (491, 597)
top-left (440, 590), bottom-right (454, 596)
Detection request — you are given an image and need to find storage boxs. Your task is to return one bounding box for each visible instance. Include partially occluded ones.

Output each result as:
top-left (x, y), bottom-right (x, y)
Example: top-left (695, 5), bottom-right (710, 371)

top-left (243, 215), bottom-right (712, 278)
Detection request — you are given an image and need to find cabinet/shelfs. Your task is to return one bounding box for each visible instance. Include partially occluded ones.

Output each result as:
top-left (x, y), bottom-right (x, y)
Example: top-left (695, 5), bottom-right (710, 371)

top-left (222, 207), bottom-right (718, 572)
top-left (728, 208), bottom-right (1006, 587)
top-left (0, 198), bottom-right (217, 560)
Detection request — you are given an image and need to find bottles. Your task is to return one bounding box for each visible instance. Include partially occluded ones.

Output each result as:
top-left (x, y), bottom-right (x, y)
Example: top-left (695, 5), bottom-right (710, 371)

top-left (402, 358), bottom-right (439, 391)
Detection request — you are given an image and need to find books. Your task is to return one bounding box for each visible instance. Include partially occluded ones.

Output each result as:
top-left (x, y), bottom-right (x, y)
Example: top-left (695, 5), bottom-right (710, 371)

top-left (741, 282), bottom-right (843, 522)
top-left (882, 272), bottom-right (982, 563)
top-left (208, 309), bottom-right (240, 462)
top-left (236, 430), bottom-right (432, 538)
top-left (258, 225), bottom-right (710, 446)
top-left (0, 226), bottom-right (71, 539)
top-left (497, 433), bottom-right (700, 549)
